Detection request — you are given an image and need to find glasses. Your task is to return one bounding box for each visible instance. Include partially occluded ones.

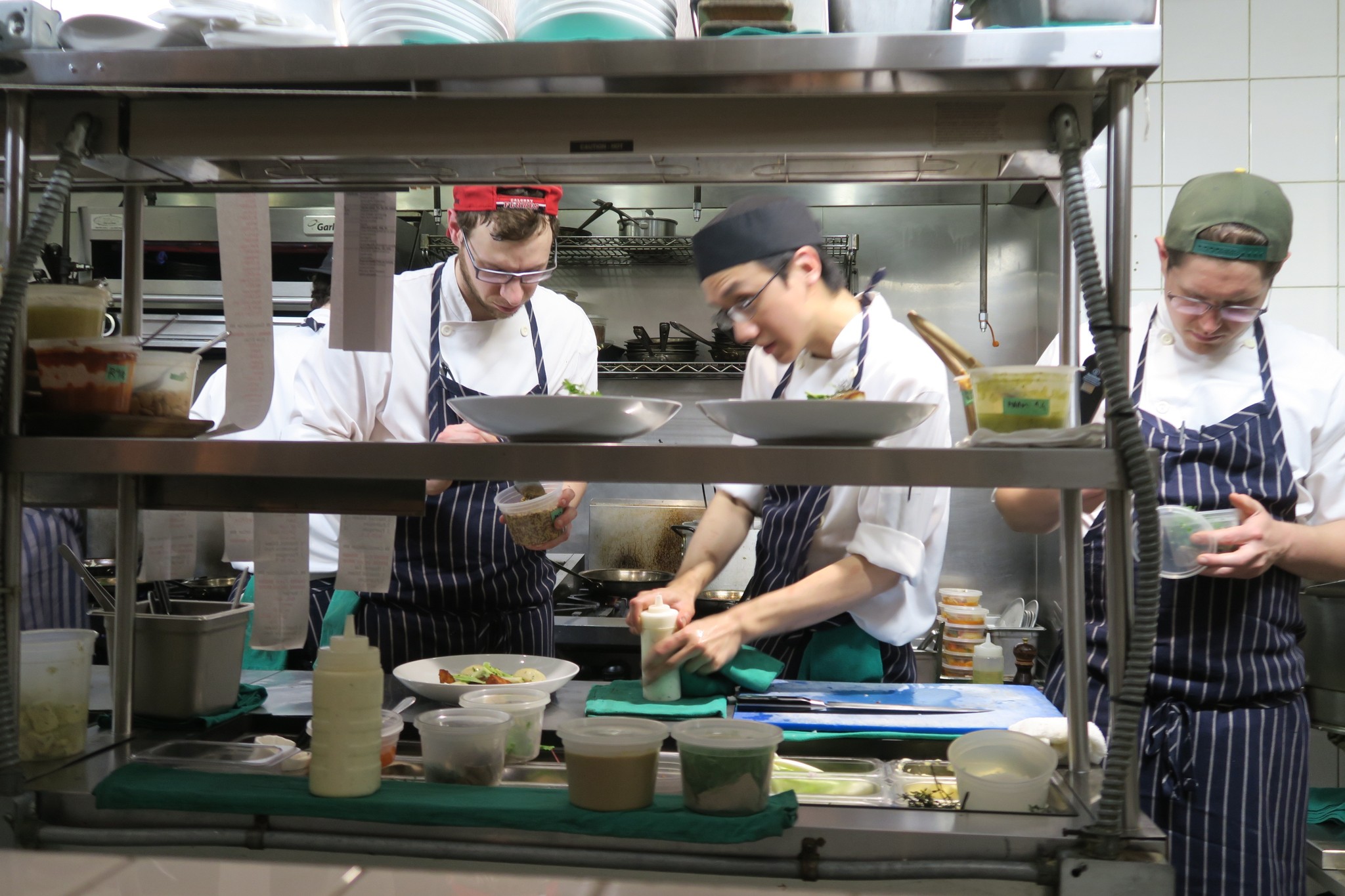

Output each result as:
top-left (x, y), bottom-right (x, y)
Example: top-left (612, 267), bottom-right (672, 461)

top-left (711, 257), bottom-right (792, 331)
top-left (1165, 266), bottom-right (1274, 322)
top-left (460, 227), bottom-right (557, 284)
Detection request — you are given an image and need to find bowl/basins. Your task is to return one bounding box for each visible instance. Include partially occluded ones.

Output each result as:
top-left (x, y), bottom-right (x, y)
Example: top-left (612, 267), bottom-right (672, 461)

top-left (304, 709), bottom-right (405, 768)
top-left (413, 706), bottom-right (511, 787)
top-left (555, 717), bottom-right (784, 817)
top-left (1163, 507), bottom-right (1241, 568)
top-left (24, 283), bottom-right (202, 417)
top-left (493, 480), bottom-right (567, 547)
top-left (946, 729), bottom-right (1058, 813)
top-left (937, 588), bottom-right (989, 674)
top-left (952, 363), bottom-right (1087, 437)
top-left (458, 688), bottom-right (552, 765)
top-left (392, 653), bottom-right (580, 704)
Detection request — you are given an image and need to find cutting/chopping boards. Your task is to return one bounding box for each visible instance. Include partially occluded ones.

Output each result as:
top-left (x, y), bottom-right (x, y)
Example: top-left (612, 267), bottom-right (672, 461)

top-left (733, 679), bottom-right (1065, 734)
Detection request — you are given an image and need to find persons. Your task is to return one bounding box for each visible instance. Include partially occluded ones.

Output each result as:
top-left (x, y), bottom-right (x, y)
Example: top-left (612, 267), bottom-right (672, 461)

top-left (988, 168), bottom-right (1345, 896)
top-left (623, 188), bottom-right (951, 683)
top-left (187, 185), bottom-right (598, 668)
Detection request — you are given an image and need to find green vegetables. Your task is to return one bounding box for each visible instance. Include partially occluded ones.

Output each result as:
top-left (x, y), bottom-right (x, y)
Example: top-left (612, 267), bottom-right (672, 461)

top-left (894, 764), bottom-right (963, 809)
top-left (562, 379), bottom-right (603, 396)
top-left (805, 392), bottom-right (828, 400)
top-left (1169, 506), bottom-right (1236, 553)
top-left (451, 662), bottom-right (514, 684)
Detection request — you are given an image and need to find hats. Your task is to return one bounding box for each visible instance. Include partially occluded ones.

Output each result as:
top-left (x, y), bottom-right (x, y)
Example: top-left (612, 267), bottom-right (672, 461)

top-left (1165, 168), bottom-right (1293, 261)
top-left (453, 185), bottom-right (563, 216)
top-left (299, 248), bottom-right (333, 273)
top-left (691, 193), bottom-right (822, 283)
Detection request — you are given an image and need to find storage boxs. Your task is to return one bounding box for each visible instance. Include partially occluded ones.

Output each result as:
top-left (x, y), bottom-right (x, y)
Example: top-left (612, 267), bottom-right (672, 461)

top-left (82, 595), bottom-right (258, 721)
top-left (407, 684), bottom-right (1109, 823)
top-left (936, 584), bottom-right (993, 680)
top-left (1153, 497), bottom-right (1242, 581)
top-left (15, 275), bottom-right (204, 431)
top-left (952, 360), bottom-right (1088, 445)
top-left (489, 480), bottom-right (576, 550)
top-left (17, 623), bottom-right (99, 768)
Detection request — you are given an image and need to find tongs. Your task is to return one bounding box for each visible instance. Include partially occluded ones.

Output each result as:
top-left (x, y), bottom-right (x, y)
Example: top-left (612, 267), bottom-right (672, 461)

top-left (58, 543), bottom-right (115, 610)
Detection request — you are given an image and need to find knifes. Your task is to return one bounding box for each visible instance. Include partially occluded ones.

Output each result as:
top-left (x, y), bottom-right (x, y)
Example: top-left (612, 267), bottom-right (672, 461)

top-left (736, 695), bottom-right (994, 713)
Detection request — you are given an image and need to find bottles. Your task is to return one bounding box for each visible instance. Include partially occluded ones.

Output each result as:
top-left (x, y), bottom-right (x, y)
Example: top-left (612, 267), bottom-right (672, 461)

top-left (973, 631), bottom-right (1005, 685)
top-left (641, 592), bottom-right (682, 702)
top-left (308, 614), bottom-right (384, 798)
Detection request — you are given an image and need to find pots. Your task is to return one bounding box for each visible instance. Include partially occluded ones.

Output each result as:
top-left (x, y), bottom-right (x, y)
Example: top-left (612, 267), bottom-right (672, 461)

top-left (543, 520), bottom-right (761, 611)
top-left (164, 576), bottom-right (236, 599)
top-left (81, 557), bottom-right (116, 576)
top-left (537, 195), bottom-right (753, 371)
top-left (95, 578), bottom-right (116, 592)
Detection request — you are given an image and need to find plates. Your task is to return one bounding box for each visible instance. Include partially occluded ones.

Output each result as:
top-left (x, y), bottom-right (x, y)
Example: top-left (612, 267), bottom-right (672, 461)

top-left (449, 392), bottom-right (940, 445)
top-left (56, 0), bottom-right (679, 49)
top-left (999, 596), bottom-right (1040, 628)
top-left (21, 412), bottom-right (214, 439)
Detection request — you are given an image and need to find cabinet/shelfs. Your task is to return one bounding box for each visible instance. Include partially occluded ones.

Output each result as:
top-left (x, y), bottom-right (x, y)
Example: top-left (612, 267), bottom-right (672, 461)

top-left (414, 223), bottom-right (864, 386)
top-left (0, 21), bottom-right (1174, 896)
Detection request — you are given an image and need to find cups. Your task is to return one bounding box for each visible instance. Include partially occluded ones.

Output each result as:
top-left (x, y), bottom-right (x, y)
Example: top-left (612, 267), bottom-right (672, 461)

top-left (20, 628), bottom-right (99, 759)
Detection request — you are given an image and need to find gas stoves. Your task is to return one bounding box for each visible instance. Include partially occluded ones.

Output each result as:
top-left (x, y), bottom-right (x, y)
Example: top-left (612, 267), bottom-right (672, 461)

top-left (88, 580), bottom-right (235, 606)
top-left (554, 586), bottom-right (631, 620)
